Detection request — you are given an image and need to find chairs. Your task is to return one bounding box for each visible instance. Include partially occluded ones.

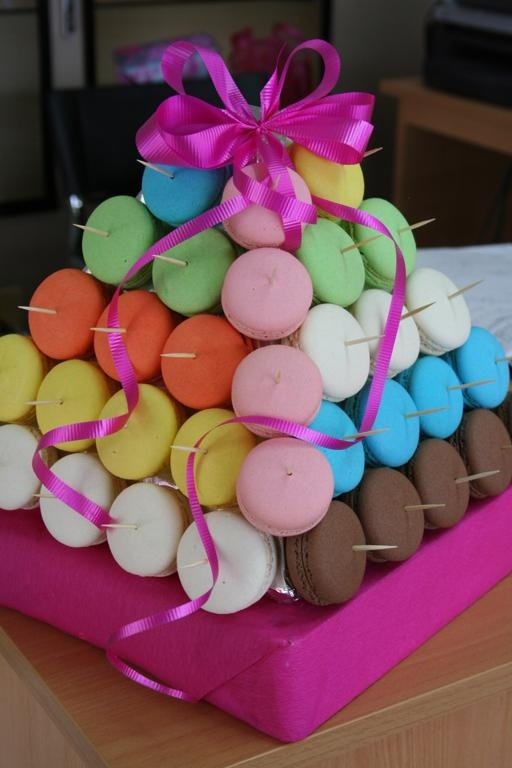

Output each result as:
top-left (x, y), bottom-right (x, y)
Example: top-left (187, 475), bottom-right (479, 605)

top-left (44, 72), bottom-right (381, 257)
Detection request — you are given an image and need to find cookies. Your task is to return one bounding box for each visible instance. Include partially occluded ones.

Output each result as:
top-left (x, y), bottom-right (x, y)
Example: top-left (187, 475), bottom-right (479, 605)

top-left (0, 103), bottom-right (511, 614)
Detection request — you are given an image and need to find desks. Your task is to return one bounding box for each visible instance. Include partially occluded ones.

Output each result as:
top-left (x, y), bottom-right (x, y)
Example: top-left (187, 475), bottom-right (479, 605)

top-left (378, 71), bottom-right (511, 246)
top-left (0, 570), bottom-right (512, 768)
